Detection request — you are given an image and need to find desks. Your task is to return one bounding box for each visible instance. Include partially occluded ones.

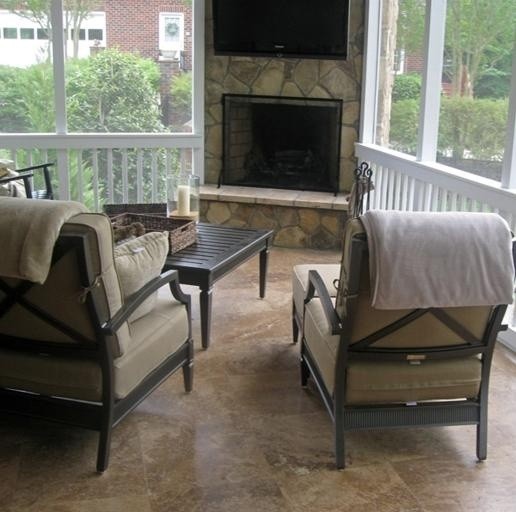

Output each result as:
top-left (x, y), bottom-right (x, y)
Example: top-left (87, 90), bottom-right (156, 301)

top-left (160, 223), bottom-right (274, 348)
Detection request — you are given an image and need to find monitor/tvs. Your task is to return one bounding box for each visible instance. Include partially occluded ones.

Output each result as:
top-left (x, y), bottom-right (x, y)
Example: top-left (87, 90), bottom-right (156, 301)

top-left (213, 0), bottom-right (350, 60)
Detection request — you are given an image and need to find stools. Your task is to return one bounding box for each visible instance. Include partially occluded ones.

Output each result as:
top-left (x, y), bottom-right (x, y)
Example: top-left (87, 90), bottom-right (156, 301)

top-left (292, 263), bottom-right (344, 343)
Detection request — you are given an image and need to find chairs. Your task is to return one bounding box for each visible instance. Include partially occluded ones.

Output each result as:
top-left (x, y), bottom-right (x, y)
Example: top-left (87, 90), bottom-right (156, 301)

top-left (1, 196), bottom-right (193, 472)
top-left (1, 162), bottom-right (56, 201)
top-left (300, 211), bottom-right (515, 470)
